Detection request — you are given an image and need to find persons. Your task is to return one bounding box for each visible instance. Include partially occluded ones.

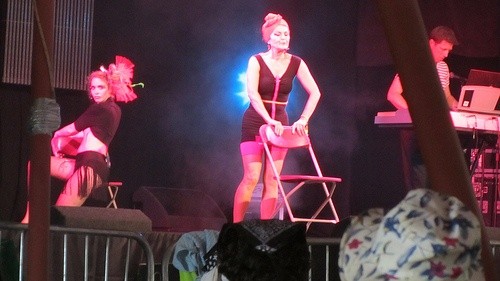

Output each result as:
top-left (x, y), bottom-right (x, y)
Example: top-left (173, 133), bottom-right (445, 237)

top-left (51, 72), bottom-right (122, 206)
top-left (387, 26), bottom-right (457, 191)
top-left (204, 187), bottom-right (487, 281)
top-left (233, 13), bottom-right (321, 223)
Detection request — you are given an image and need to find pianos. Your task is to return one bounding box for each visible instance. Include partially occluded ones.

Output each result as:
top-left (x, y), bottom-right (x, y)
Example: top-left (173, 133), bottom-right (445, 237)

top-left (373, 108), bottom-right (500, 181)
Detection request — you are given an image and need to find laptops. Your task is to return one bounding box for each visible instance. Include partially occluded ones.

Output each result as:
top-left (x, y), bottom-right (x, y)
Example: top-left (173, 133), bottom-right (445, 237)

top-left (457, 86), bottom-right (500, 110)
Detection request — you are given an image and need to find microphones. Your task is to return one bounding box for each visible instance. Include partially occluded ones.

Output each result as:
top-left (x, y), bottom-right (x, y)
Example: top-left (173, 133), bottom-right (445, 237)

top-left (132, 82), bottom-right (144, 88)
top-left (449, 72), bottom-right (462, 81)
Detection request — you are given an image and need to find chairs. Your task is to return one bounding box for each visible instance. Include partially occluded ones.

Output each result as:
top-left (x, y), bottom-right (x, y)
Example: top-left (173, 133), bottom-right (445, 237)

top-left (52, 136), bottom-right (123, 211)
top-left (259, 124), bottom-right (344, 235)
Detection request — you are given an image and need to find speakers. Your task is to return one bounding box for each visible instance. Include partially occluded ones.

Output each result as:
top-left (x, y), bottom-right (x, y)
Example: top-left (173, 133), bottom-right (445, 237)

top-left (51, 186), bottom-right (230, 231)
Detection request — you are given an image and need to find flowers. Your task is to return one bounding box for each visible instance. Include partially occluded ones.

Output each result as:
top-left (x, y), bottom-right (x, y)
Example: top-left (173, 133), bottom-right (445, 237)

top-left (105, 55), bottom-right (144, 105)
